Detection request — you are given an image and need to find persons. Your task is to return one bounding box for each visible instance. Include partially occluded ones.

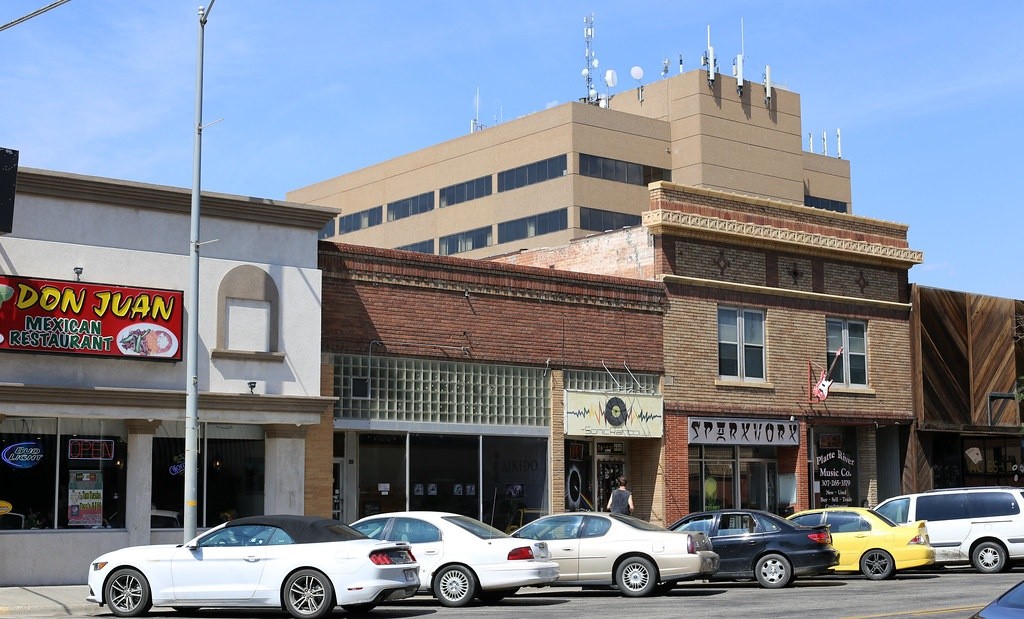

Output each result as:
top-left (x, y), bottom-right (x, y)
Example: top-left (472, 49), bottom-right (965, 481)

top-left (607, 476), bottom-right (634, 516)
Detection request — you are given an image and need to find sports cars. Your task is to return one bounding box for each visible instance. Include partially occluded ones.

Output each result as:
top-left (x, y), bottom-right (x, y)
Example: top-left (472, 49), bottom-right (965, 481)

top-left (84, 513), bottom-right (423, 619)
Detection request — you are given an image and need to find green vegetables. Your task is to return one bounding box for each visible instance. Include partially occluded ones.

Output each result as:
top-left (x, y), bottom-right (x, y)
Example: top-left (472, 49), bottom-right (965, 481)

top-left (120, 329), bottom-right (151, 352)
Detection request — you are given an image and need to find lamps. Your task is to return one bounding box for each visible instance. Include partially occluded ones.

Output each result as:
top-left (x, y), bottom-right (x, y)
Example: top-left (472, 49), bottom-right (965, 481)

top-left (248, 382), bottom-right (256, 394)
top-left (73, 267), bottom-right (83, 282)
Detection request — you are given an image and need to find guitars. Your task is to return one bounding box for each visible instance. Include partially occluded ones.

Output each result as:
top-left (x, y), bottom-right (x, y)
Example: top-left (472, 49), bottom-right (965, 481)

top-left (812, 346), bottom-right (844, 401)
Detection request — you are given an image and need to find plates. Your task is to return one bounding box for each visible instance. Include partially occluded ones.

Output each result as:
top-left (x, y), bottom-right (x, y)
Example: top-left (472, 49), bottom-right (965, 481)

top-left (116, 323), bottom-right (179, 358)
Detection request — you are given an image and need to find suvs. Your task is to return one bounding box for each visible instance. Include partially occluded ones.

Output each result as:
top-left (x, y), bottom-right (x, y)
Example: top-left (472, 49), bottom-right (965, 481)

top-left (868, 485), bottom-right (1024, 574)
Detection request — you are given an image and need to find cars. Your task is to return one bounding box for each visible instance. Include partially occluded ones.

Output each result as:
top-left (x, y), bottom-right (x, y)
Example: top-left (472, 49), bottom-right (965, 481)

top-left (664, 508), bottom-right (841, 589)
top-left (508, 511), bottom-right (720, 598)
top-left (782, 505), bottom-right (938, 580)
top-left (347, 511), bottom-right (562, 608)
